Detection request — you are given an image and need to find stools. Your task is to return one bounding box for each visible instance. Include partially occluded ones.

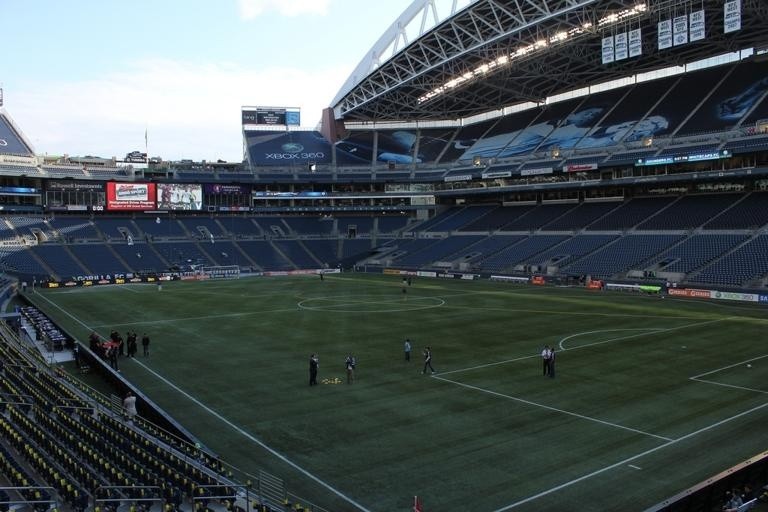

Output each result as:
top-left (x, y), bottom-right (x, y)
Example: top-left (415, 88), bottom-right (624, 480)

top-left (1, 304), bottom-right (328, 512)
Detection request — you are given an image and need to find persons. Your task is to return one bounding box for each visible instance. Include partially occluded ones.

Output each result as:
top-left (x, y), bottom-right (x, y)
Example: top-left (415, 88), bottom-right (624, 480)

top-left (345, 353), bottom-right (355, 384)
top-left (404, 339), bottom-right (411, 362)
top-left (722, 482), bottom-right (757, 512)
top-left (62, 198), bottom-right (104, 216)
top-left (158, 184), bottom-right (202, 211)
top-left (310, 353), bottom-right (320, 387)
top-left (10, 279), bottom-right (50, 294)
top-left (542, 345), bottom-right (551, 376)
top-left (661, 278), bottom-right (678, 295)
top-left (29, 313), bottom-right (65, 351)
top-left (123, 391), bottom-right (137, 416)
top-left (643, 270), bottom-right (657, 280)
top-left (71, 329), bottom-right (151, 373)
top-left (400, 273), bottom-right (417, 302)
top-left (319, 271), bottom-right (325, 282)
top-left (421, 346), bottom-right (436, 374)
top-left (549, 347), bottom-right (556, 378)
